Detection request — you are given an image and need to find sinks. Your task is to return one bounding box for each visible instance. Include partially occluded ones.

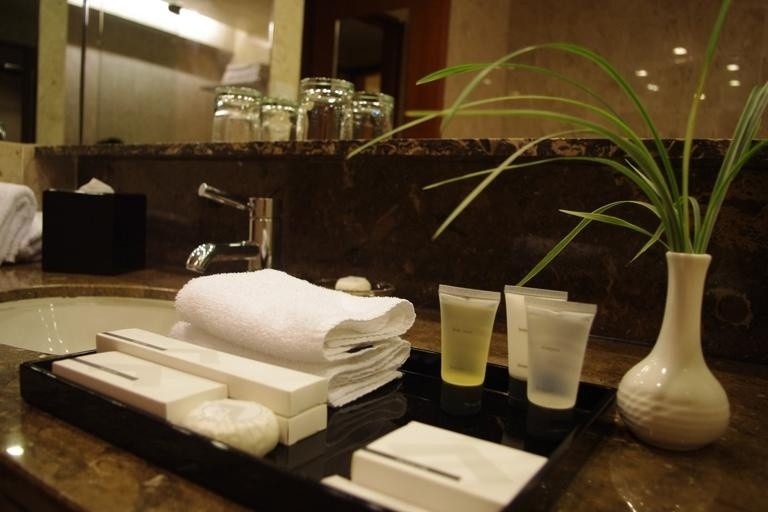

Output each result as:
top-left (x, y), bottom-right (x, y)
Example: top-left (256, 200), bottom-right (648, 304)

top-left (0, 283), bottom-right (183, 357)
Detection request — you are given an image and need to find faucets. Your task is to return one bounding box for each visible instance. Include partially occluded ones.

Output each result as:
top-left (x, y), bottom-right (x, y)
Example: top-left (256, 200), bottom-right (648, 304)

top-left (184, 182), bottom-right (274, 273)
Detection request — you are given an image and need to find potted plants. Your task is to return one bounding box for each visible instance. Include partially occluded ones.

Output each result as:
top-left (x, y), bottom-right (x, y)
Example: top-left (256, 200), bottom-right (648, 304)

top-left (347, 0), bottom-right (768, 454)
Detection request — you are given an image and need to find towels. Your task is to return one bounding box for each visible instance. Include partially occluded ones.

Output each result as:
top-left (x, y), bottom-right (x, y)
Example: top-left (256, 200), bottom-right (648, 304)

top-left (19, 210), bottom-right (43, 261)
top-left (173, 269), bottom-right (417, 364)
top-left (0, 180), bottom-right (37, 267)
top-left (170, 320), bottom-right (412, 407)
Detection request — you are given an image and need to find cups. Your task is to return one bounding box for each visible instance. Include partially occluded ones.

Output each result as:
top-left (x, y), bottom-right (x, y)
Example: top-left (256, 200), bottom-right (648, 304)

top-left (261, 102), bottom-right (296, 143)
top-left (353, 90), bottom-right (396, 140)
top-left (296, 77), bottom-right (355, 142)
top-left (524, 296), bottom-right (598, 409)
top-left (211, 87), bottom-right (264, 143)
top-left (438, 284), bottom-right (500, 387)
top-left (504, 285), bottom-right (567, 380)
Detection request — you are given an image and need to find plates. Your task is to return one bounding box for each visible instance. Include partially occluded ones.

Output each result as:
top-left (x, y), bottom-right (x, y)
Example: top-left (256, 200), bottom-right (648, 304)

top-left (18, 345), bottom-right (616, 511)
